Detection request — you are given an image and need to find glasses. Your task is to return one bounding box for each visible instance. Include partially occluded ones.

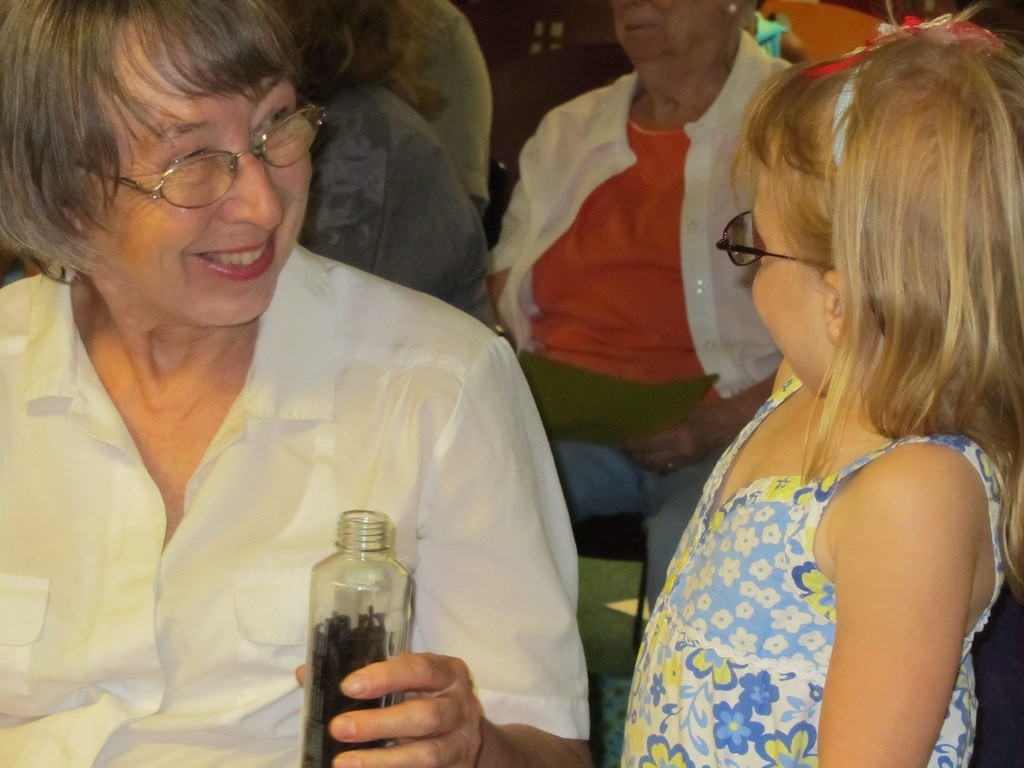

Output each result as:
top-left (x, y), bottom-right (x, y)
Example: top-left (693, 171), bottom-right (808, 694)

top-left (716, 212), bottom-right (819, 266)
top-left (74, 95), bottom-right (327, 208)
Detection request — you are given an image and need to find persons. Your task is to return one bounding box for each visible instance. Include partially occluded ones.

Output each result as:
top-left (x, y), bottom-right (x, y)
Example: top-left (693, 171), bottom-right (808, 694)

top-left (489, 0), bottom-right (791, 612)
top-left (0, 0), bottom-right (592, 768)
top-left (620, 14), bottom-right (1024, 768)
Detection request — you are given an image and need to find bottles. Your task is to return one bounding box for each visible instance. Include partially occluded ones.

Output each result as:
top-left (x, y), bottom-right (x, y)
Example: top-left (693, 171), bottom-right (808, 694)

top-left (301, 509), bottom-right (413, 768)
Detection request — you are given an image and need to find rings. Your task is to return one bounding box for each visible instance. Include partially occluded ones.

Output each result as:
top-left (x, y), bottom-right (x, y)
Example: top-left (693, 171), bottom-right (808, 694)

top-left (668, 463), bottom-right (673, 471)
